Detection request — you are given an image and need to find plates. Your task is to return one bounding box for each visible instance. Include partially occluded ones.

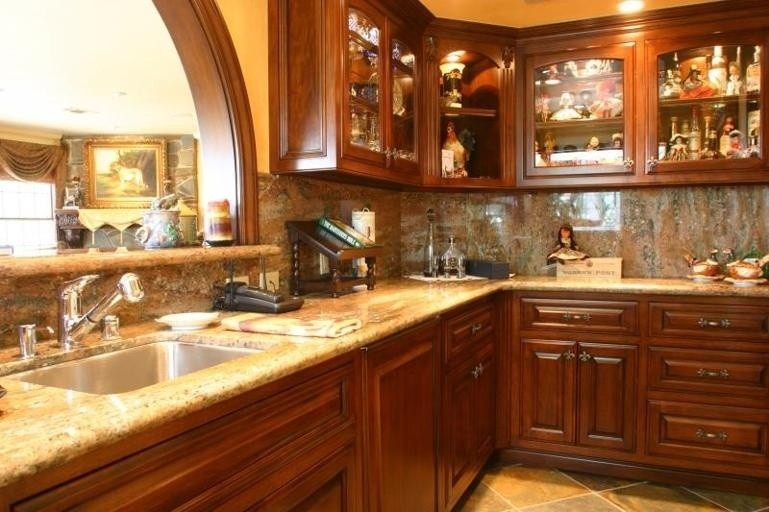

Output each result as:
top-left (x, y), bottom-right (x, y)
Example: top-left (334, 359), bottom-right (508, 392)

top-left (157, 312), bottom-right (221, 330)
top-left (685, 274), bottom-right (767, 289)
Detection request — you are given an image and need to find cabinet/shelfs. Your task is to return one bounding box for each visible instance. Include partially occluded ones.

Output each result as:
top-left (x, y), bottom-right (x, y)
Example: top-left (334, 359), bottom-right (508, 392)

top-left (640, 289), bottom-right (769, 501)
top-left (0, 338), bottom-right (362, 511)
top-left (428, 14), bottom-right (520, 194)
top-left (361, 314), bottom-right (450, 511)
top-left (507, 286), bottom-right (640, 481)
top-left (521, 0), bottom-right (769, 192)
top-left (262, 0), bottom-right (435, 194)
top-left (438, 282), bottom-right (506, 512)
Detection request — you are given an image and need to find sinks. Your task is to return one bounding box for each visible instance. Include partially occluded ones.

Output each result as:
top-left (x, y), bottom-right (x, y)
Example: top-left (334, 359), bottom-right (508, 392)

top-left (0, 332), bottom-right (275, 397)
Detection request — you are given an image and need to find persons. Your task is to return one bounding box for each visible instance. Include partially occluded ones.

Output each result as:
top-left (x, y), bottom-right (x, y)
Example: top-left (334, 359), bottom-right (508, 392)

top-left (545, 222), bottom-right (590, 261)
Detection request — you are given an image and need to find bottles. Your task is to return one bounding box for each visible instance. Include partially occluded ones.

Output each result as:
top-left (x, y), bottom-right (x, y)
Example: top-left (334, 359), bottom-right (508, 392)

top-left (424, 208), bottom-right (466, 280)
top-left (349, 8), bottom-right (417, 164)
top-left (439, 60), bottom-right (465, 118)
top-left (658, 44), bottom-right (762, 161)
top-left (532, 58), bottom-right (625, 165)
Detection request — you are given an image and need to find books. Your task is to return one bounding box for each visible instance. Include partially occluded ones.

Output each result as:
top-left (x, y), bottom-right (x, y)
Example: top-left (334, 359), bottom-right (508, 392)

top-left (315, 216), bottom-right (377, 250)
top-left (318, 225), bottom-right (351, 250)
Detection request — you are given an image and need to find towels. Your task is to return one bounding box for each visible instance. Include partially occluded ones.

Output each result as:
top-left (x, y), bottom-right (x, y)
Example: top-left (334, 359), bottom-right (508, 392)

top-left (218, 306), bottom-right (363, 340)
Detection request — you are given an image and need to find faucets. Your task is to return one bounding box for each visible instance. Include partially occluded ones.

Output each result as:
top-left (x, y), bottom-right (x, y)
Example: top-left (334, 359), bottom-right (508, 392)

top-left (57, 271), bottom-right (146, 347)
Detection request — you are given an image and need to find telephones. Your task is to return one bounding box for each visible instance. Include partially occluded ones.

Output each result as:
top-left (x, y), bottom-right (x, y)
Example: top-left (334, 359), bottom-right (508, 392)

top-left (211, 257), bottom-right (304, 314)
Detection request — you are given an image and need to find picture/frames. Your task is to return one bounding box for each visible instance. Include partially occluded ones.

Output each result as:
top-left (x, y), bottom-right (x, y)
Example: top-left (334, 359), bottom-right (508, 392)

top-left (83, 138), bottom-right (170, 210)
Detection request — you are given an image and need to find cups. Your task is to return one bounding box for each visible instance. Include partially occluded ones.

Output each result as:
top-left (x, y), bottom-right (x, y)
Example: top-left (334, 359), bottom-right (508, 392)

top-left (134, 197), bottom-right (236, 247)
top-left (692, 259), bottom-right (763, 279)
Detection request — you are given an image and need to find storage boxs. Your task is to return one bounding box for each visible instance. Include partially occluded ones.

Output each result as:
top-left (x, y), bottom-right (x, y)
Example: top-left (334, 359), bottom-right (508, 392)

top-left (462, 258), bottom-right (511, 280)
top-left (555, 256), bottom-right (624, 278)
